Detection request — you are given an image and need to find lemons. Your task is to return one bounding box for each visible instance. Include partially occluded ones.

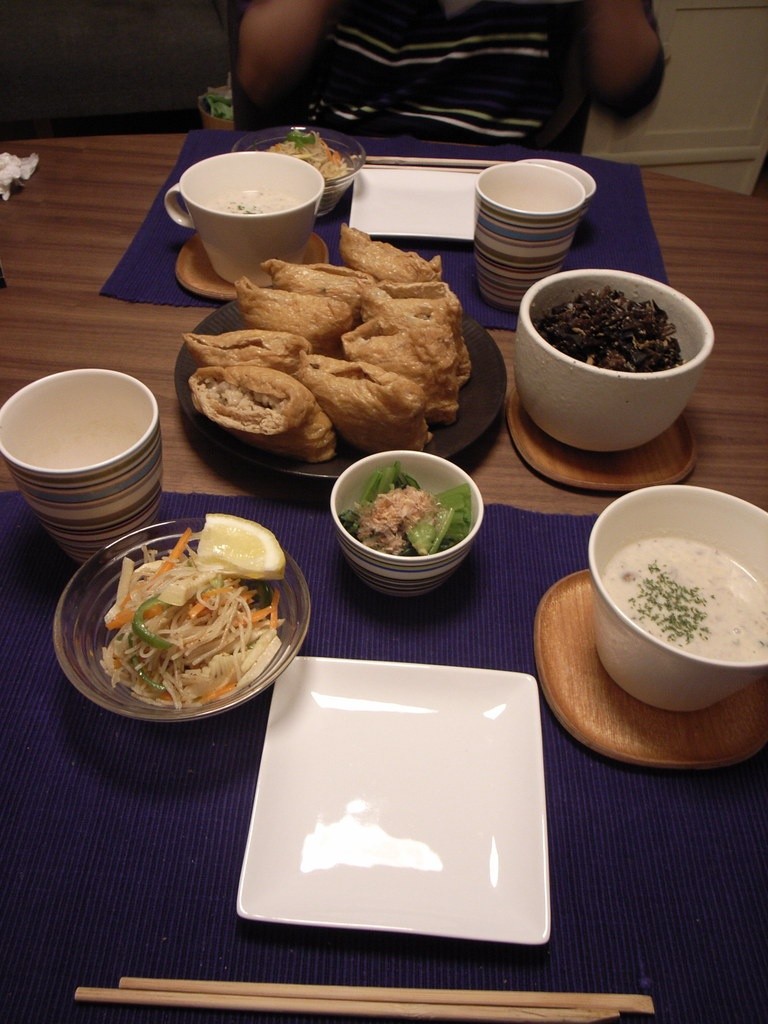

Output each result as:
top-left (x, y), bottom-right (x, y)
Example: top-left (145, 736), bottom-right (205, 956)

top-left (194, 513), bottom-right (284, 580)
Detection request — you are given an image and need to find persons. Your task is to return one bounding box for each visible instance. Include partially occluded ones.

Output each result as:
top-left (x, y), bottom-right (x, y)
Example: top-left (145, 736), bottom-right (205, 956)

top-left (229, 0), bottom-right (665, 156)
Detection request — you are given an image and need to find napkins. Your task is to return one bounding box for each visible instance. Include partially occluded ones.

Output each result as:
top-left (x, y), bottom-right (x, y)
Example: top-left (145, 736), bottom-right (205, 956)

top-left (0, 149), bottom-right (40, 200)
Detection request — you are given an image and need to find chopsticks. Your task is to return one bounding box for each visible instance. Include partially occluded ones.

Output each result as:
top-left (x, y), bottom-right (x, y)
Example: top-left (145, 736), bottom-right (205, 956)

top-left (76, 978), bottom-right (655, 1024)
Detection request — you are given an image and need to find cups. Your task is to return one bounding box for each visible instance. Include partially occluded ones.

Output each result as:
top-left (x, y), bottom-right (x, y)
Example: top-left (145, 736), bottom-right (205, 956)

top-left (0, 368), bottom-right (164, 565)
top-left (476, 163), bottom-right (586, 314)
top-left (165, 151), bottom-right (325, 287)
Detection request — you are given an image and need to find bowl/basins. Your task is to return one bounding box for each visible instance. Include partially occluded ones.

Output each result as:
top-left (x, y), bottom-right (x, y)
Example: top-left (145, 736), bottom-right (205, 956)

top-left (331, 451), bottom-right (485, 598)
top-left (52, 516), bottom-right (311, 723)
top-left (232, 127), bottom-right (366, 217)
top-left (515, 268), bottom-right (714, 451)
top-left (196, 86), bottom-right (234, 129)
top-left (516, 159), bottom-right (596, 216)
top-left (590, 484), bottom-right (768, 710)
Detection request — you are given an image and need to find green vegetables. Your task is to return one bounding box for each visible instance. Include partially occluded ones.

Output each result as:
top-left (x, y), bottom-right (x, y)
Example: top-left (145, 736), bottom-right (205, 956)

top-left (286, 130), bottom-right (316, 149)
top-left (338, 460), bottom-right (473, 556)
top-left (202, 93), bottom-right (233, 121)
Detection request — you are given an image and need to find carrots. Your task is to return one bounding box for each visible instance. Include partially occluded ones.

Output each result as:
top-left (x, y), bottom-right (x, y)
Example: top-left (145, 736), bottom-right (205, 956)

top-left (276, 133), bottom-right (342, 166)
top-left (106, 526), bottom-right (278, 703)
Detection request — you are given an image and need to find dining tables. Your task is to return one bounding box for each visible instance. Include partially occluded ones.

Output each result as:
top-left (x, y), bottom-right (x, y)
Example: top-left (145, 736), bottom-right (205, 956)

top-left (0, 129), bottom-right (767, 1024)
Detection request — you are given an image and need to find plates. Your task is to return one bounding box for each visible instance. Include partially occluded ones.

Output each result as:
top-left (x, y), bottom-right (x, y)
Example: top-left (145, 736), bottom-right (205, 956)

top-left (350, 165), bottom-right (480, 240)
top-left (237, 656), bottom-right (550, 944)
top-left (174, 299), bottom-right (507, 478)
